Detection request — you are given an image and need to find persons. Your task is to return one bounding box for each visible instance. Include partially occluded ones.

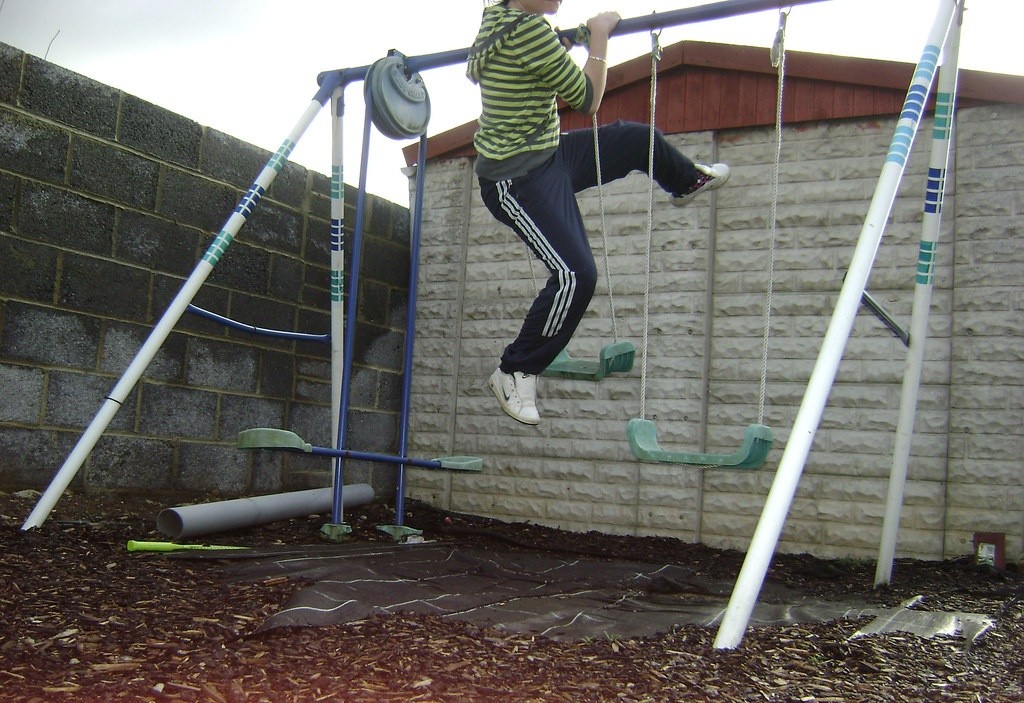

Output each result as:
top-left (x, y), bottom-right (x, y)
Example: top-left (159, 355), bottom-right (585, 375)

top-left (462, 1), bottom-right (731, 425)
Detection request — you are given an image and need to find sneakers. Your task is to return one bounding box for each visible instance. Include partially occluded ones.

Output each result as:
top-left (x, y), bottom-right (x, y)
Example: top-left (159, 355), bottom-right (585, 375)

top-left (487, 367), bottom-right (542, 425)
top-left (668, 163), bottom-right (731, 207)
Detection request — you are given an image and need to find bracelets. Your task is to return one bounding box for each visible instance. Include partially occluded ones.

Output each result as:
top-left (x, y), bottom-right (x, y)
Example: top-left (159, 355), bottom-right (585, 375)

top-left (588, 55), bottom-right (607, 64)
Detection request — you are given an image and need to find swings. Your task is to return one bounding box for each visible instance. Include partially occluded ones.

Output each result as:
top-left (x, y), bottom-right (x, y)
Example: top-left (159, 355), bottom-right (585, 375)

top-left (622, 9), bottom-right (792, 472)
top-left (469, 35), bottom-right (636, 384)
